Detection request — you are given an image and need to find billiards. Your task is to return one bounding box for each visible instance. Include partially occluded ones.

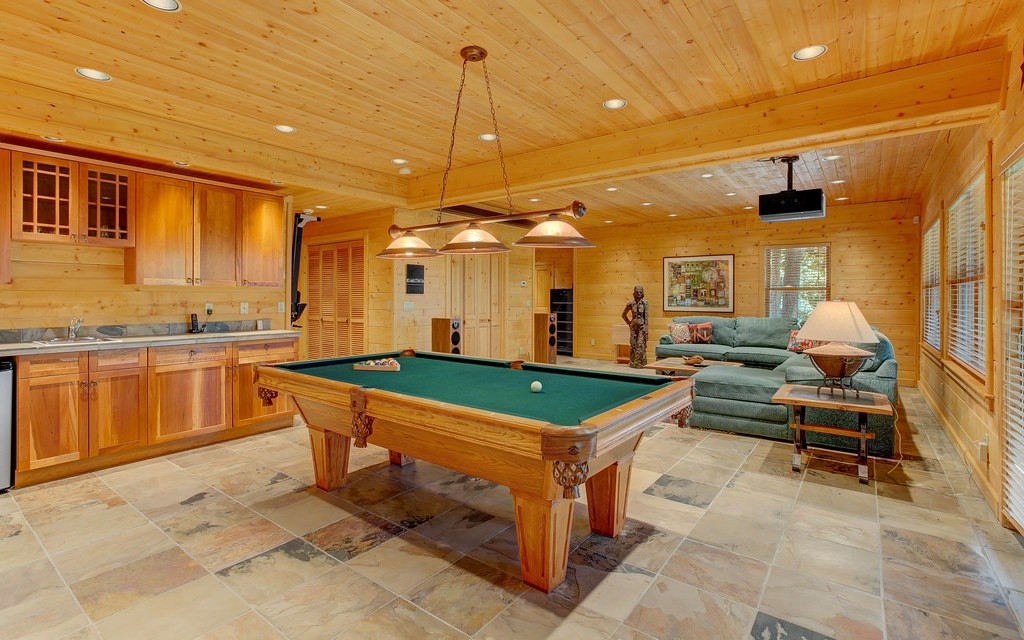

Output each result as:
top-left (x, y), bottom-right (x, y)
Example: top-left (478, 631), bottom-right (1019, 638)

top-left (530, 381), bottom-right (543, 392)
top-left (355, 357), bottom-right (398, 367)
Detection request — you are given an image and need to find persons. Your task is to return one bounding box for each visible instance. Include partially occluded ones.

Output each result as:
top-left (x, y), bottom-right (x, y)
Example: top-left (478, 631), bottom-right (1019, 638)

top-left (622, 286), bottom-right (648, 369)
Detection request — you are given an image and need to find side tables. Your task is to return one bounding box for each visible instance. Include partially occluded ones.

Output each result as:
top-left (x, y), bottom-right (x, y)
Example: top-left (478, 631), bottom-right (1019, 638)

top-left (772, 384), bottom-right (894, 484)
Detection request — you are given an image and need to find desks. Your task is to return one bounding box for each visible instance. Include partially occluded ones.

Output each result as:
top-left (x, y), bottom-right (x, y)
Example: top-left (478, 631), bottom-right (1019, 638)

top-left (252, 350), bottom-right (696, 594)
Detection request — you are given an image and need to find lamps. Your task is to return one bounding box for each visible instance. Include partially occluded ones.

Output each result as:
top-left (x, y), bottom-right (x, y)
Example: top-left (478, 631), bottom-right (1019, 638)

top-left (377, 46), bottom-right (598, 259)
top-left (796, 301), bottom-right (880, 400)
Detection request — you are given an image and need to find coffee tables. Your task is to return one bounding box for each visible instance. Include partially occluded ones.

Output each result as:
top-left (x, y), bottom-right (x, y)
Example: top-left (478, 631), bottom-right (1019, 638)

top-left (644, 357), bottom-right (745, 375)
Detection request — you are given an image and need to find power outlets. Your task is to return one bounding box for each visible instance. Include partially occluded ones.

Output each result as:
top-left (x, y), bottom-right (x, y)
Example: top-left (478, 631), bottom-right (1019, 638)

top-left (913, 216), bottom-right (919, 224)
top-left (204, 303), bottom-right (213, 315)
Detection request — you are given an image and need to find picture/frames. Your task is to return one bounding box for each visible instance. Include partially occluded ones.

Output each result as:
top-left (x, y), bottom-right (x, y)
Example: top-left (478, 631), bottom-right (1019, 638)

top-left (663, 254), bottom-right (735, 313)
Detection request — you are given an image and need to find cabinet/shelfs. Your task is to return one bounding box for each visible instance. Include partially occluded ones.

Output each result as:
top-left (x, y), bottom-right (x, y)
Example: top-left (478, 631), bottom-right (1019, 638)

top-left (13, 347), bottom-right (149, 487)
top-left (123, 173), bottom-right (240, 287)
top-left (240, 190), bottom-right (284, 288)
top-left (149, 342), bottom-right (233, 458)
top-left (11, 149), bottom-right (136, 249)
top-left (550, 289), bottom-right (573, 357)
top-left (613, 323), bottom-right (630, 364)
top-left (0, 147), bottom-right (11, 283)
top-left (233, 338), bottom-right (297, 438)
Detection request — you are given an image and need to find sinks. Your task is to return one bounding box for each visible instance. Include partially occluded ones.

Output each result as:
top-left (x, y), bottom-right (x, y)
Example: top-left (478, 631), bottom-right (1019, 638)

top-left (32, 336), bottom-right (121, 346)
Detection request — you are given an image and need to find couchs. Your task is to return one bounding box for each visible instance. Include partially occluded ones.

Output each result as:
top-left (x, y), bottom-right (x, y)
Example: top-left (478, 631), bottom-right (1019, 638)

top-left (656, 316), bottom-right (897, 458)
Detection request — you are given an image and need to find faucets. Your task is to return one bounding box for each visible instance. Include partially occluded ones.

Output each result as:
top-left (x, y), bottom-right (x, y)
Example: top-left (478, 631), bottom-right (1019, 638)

top-left (68, 317), bottom-right (84, 339)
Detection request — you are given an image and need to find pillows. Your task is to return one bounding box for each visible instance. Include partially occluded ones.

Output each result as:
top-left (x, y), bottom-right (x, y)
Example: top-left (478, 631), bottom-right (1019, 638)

top-left (787, 330), bottom-right (814, 353)
top-left (688, 322), bottom-right (712, 343)
top-left (668, 323), bottom-right (691, 344)
top-left (814, 340), bottom-right (831, 347)
